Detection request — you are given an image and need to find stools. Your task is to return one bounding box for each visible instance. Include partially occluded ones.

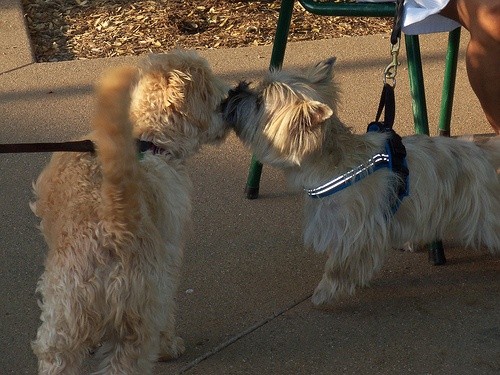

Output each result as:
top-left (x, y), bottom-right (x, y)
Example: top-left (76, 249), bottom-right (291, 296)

top-left (244, 0), bottom-right (463, 264)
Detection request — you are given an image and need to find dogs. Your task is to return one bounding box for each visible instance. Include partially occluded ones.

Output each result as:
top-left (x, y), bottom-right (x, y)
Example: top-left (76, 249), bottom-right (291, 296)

top-left (30, 48), bottom-right (230, 375)
top-left (217, 56), bottom-right (500, 308)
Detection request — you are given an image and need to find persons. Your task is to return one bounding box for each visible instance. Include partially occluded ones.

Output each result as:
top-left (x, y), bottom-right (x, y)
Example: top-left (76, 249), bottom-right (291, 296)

top-left (400, 0), bottom-right (500, 136)
